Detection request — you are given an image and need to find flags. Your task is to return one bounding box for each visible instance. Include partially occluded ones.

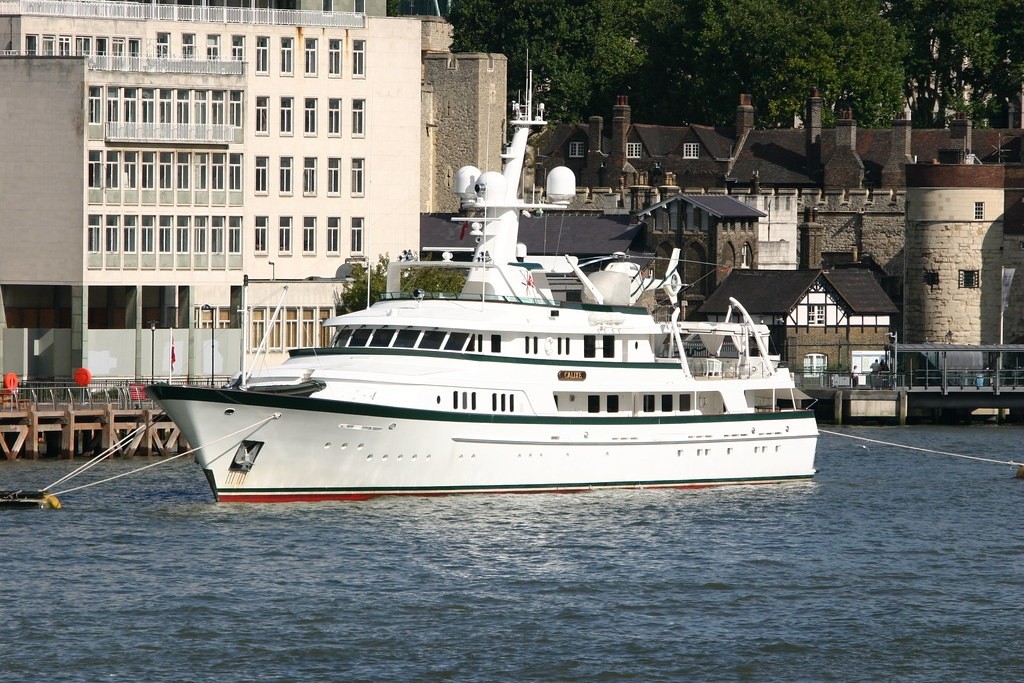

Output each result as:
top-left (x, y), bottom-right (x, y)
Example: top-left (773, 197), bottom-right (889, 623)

top-left (1001, 268), bottom-right (1016, 312)
top-left (172, 336), bottom-right (176, 372)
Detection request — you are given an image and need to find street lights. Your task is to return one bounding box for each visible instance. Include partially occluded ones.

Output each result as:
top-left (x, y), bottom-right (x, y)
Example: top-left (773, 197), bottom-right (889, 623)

top-left (202, 304), bottom-right (215, 388)
top-left (146, 319), bottom-right (159, 384)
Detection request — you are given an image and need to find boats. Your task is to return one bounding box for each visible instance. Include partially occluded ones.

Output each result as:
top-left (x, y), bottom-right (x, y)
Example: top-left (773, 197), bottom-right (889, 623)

top-left (144, 45), bottom-right (820, 503)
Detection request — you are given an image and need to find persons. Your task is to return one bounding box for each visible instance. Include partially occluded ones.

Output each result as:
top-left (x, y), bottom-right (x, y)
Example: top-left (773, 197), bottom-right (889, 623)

top-left (851, 365), bottom-right (858, 387)
top-left (870, 359), bottom-right (895, 390)
